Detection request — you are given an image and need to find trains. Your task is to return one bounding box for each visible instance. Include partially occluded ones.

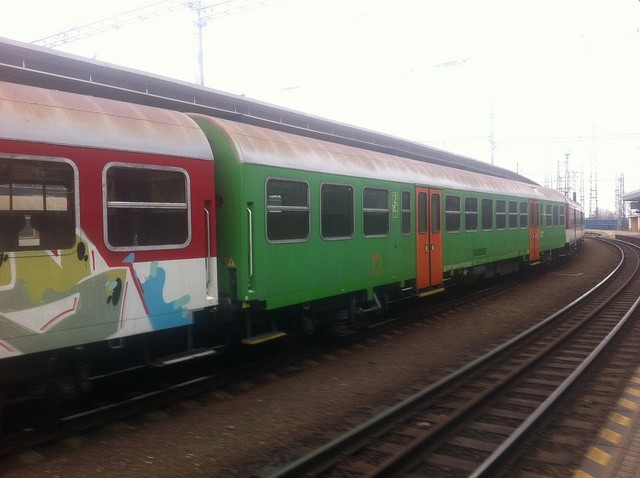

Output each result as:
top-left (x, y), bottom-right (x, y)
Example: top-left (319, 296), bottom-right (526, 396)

top-left (0, 37), bottom-right (585, 454)
